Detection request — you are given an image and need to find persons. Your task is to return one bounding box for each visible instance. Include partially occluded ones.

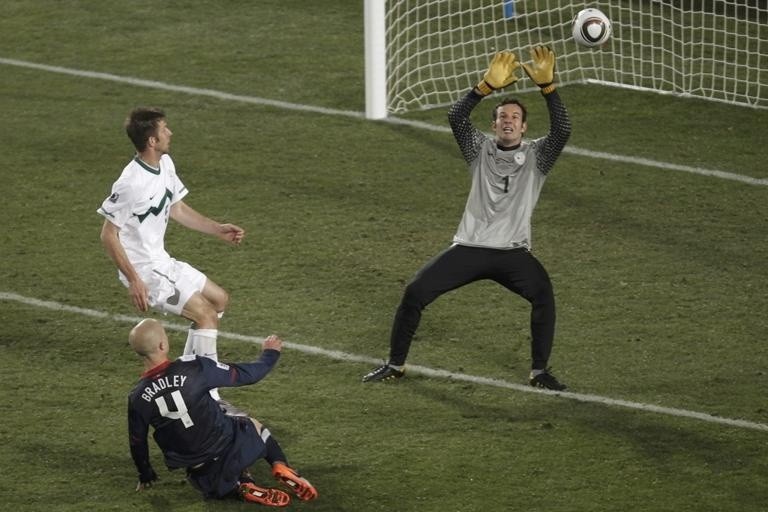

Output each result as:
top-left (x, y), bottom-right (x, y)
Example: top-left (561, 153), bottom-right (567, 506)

top-left (360, 45), bottom-right (573, 391)
top-left (127, 318), bottom-right (317, 507)
top-left (95, 106), bottom-right (248, 418)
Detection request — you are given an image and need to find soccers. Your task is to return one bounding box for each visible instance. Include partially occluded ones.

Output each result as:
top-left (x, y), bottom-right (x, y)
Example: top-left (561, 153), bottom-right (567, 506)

top-left (570, 7), bottom-right (612, 48)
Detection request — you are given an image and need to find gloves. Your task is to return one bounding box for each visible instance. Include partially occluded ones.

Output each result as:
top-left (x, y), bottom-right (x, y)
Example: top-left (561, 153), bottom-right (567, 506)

top-left (523, 44), bottom-right (555, 95)
top-left (478, 51), bottom-right (521, 96)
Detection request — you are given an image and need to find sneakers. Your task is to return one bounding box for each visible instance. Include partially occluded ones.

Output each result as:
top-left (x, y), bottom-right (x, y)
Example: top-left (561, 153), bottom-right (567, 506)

top-left (272, 463), bottom-right (319, 502)
top-left (529, 372), bottom-right (567, 390)
top-left (362, 365), bottom-right (406, 383)
top-left (236, 483), bottom-right (290, 507)
